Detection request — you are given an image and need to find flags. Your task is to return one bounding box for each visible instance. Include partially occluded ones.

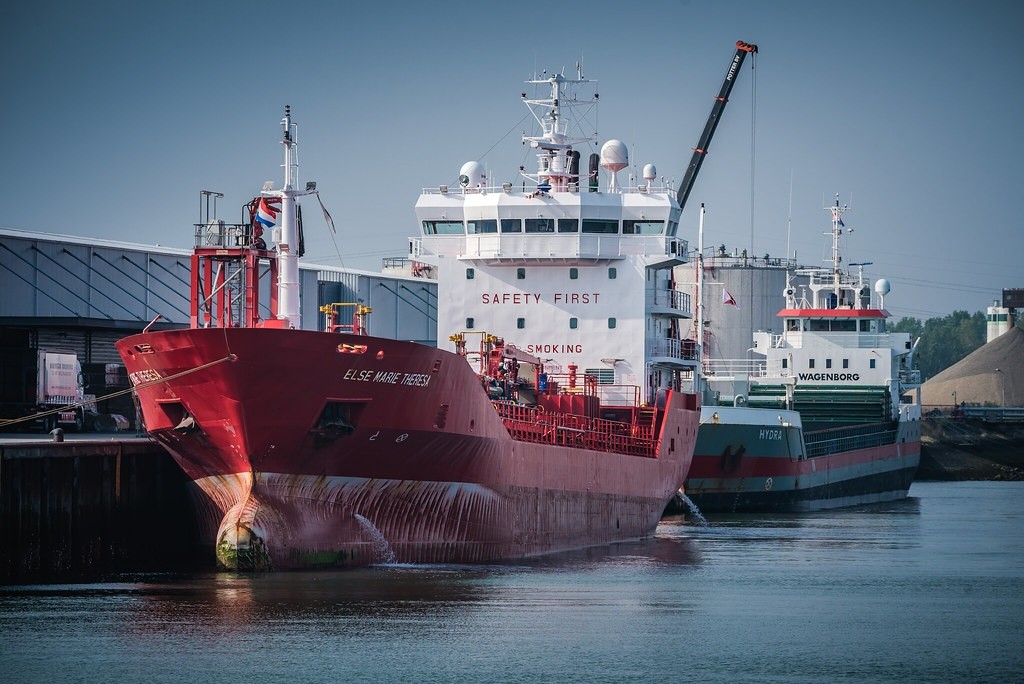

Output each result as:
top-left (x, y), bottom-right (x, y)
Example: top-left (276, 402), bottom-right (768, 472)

top-left (255, 198), bottom-right (277, 228)
top-left (722, 288), bottom-right (737, 306)
top-left (832, 211), bottom-right (845, 227)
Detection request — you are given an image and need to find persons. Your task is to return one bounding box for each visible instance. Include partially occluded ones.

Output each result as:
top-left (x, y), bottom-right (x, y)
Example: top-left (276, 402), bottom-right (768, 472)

top-left (778, 414), bottom-right (783, 424)
top-left (507, 358), bottom-right (520, 381)
top-left (712, 411), bottom-right (720, 424)
top-left (497, 362), bottom-right (507, 389)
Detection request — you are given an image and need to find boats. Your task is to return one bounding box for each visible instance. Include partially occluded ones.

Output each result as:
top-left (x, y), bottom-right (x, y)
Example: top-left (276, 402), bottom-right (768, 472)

top-left (658, 190), bottom-right (924, 514)
top-left (114, 59), bottom-right (710, 572)
